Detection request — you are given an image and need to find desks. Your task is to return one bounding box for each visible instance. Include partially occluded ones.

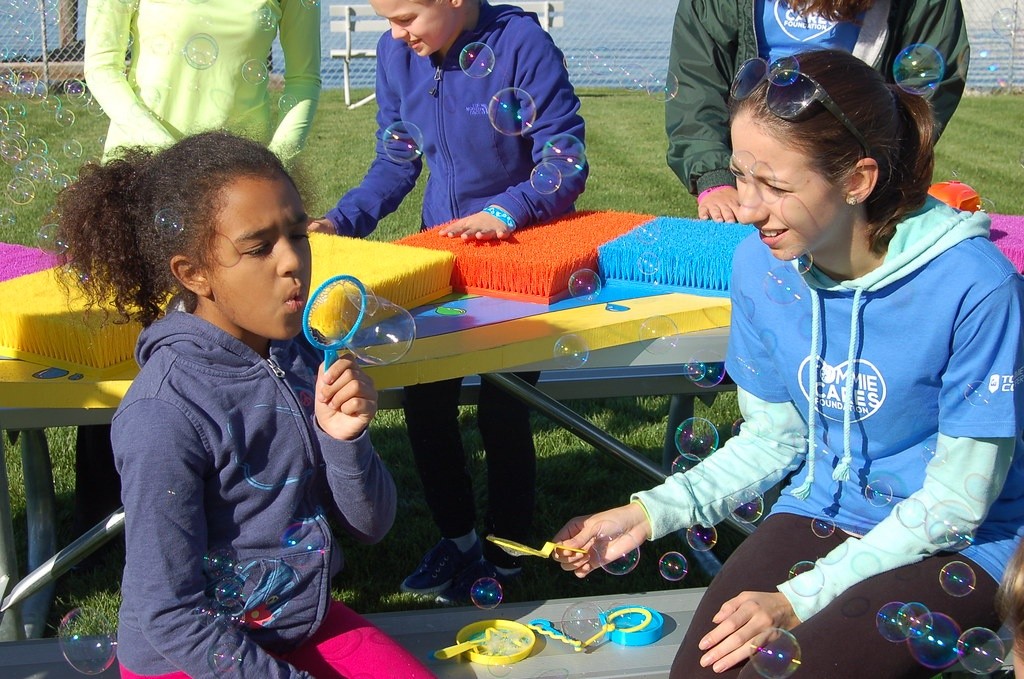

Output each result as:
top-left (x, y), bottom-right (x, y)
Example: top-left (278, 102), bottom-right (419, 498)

top-left (1, 290), bottom-right (732, 649)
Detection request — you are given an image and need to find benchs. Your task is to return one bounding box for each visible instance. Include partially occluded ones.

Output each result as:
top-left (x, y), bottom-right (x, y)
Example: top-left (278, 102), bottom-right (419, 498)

top-left (330, 2), bottom-right (563, 109)
top-left (0, 325), bottom-right (1012, 679)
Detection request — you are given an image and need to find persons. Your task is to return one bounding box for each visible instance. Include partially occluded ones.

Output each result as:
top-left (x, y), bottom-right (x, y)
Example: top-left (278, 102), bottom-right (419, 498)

top-left (665, 1), bottom-right (971, 225)
top-left (61, 0), bottom-right (321, 565)
top-left (551, 49), bottom-right (1024, 678)
top-left (306, 0), bottom-right (589, 606)
top-left (47, 131), bottom-right (439, 679)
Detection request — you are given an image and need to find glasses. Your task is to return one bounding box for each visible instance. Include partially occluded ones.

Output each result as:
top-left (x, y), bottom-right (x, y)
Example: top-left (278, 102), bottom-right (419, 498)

top-left (730, 57), bottom-right (872, 159)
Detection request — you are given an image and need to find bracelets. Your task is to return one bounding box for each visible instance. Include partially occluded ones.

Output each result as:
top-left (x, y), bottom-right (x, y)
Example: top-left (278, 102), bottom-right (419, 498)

top-left (697, 185), bottom-right (735, 204)
top-left (483, 206), bottom-right (518, 232)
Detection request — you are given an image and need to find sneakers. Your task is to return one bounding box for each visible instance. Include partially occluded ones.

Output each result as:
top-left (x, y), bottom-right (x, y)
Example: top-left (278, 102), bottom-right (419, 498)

top-left (400, 535), bottom-right (522, 605)
